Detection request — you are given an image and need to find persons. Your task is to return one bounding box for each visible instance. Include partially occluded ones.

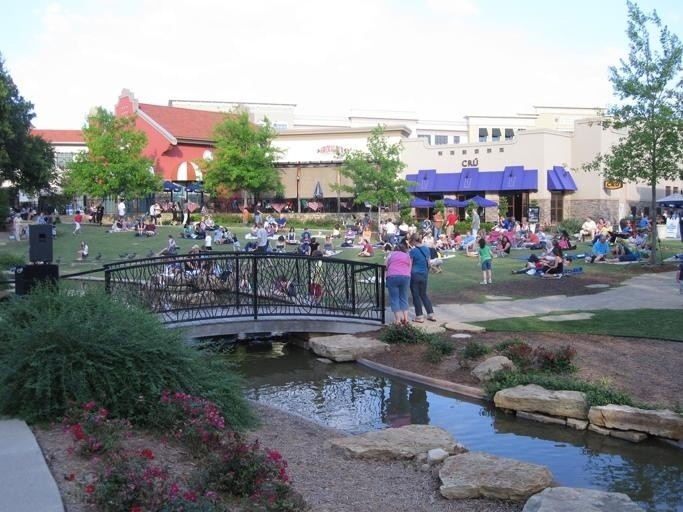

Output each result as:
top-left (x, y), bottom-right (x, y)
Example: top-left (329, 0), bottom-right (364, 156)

top-left (385, 381), bottom-right (412, 428)
top-left (341, 209), bottom-right (462, 322)
top-left (12, 197), bottom-right (89, 258)
top-left (181, 204), bottom-right (241, 252)
top-left (409, 387), bottom-right (430, 425)
top-left (577, 209), bottom-right (682, 262)
top-left (242, 199), bottom-right (341, 305)
top-left (89, 200), bottom-right (194, 237)
top-left (459, 207), bottom-right (573, 285)
top-left (165, 234), bottom-right (233, 281)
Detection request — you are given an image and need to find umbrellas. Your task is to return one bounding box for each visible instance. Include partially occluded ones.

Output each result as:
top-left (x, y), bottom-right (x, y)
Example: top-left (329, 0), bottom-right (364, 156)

top-left (160, 181), bottom-right (181, 199)
top-left (185, 181), bottom-right (208, 204)
